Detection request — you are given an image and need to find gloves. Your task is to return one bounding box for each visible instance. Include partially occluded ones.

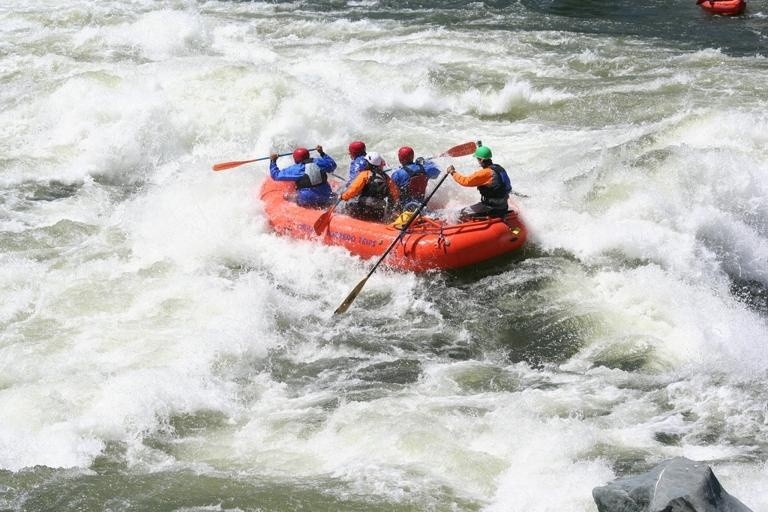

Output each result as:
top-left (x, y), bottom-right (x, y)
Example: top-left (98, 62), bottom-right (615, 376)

top-left (416, 157), bottom-right (425, 166)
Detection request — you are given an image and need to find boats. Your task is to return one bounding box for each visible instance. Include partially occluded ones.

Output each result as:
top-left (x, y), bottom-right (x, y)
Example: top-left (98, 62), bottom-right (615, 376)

top-left (697, 0), bottom-right (746, 15)
top-left (257, 167), bottom-right (529, 270)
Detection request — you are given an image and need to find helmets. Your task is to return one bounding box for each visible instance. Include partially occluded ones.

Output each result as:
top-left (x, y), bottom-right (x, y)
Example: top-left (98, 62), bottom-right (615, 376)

top-left (473, 146), bottom-right (492, 160)
top-left (348, 141), bottom-right (365, 160)
top-left (293, 148), bottom-right (309, 163)
top-left (397, 146), bottom-right (415, 164)
top-left (364, 151), bottom-right (382, 166)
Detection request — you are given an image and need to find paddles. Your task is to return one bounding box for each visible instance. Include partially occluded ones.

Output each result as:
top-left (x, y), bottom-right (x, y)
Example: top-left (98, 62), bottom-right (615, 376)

top-left (333, 171), bottom-right (450, 318)
top-left (315, 197), bottom-right (342, 237)
top-left (214, 148), bottom-right (316, 170)
top-left (384, 142), bottom-right (478, 172)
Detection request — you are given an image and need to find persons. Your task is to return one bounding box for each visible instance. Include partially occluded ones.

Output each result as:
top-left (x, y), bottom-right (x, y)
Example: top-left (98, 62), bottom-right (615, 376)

top-left (445, 146), bottom-right (509, 224)
top-left (386, 145), bottom-right (443, 217)
top-left (336, 150), bottom-right (401, 222)
top-left (343, 140), bottom-right (371, 187)
top-left (268, 143), bottom-right (338, 207)
